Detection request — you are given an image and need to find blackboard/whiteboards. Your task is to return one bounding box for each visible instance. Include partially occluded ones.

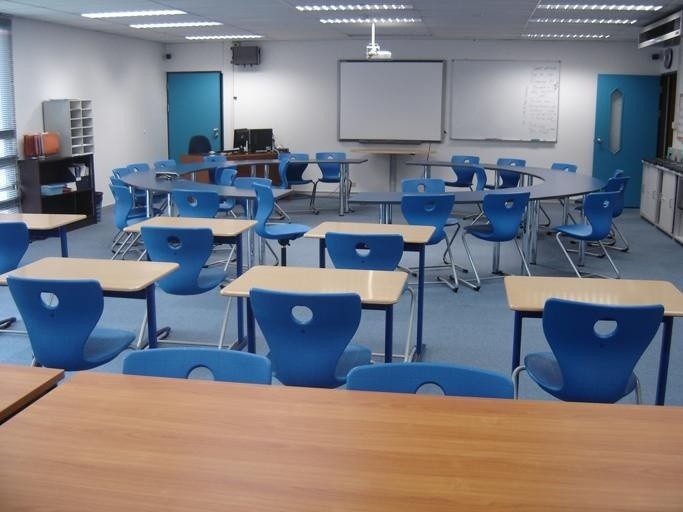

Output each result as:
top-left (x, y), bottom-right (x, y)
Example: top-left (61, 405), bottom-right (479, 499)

top-left (450, 59), bottom-right (559, 142)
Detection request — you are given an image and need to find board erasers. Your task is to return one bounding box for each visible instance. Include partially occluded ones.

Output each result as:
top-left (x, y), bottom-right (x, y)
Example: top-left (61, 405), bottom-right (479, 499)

top-left (531, 139), bottom-right (539, 141)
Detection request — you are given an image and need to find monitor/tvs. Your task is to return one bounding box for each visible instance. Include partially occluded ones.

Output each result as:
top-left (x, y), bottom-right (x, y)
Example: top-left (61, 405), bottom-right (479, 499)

top-left (248, 128), bottom-right (272, 153)
top-left (234, 128), bottom-right (247, 150)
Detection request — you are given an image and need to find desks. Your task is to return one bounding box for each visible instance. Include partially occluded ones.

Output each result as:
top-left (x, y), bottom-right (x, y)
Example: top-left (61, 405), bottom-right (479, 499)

top-left (351, 149), bottom-right (436, 191)
top-left (504, 276), bottom-right (683, 405)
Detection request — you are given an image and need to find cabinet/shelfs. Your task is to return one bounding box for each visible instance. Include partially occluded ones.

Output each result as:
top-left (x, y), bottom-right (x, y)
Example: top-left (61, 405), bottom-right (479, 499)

top-left (639, 160), bottom-right (683, 246)
top-left (44, 100), bottom-right (95, 157)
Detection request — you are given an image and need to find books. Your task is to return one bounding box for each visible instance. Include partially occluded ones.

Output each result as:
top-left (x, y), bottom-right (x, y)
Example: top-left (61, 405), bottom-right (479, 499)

top-left (23, 132), bottom-right (59, 159)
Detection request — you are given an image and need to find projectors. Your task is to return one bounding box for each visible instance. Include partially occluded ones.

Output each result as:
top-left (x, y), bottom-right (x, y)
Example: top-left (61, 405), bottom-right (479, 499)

top-left (364, 52), bottom-right (390, 60)
top-left (230, 46), bottom-right (264, 65)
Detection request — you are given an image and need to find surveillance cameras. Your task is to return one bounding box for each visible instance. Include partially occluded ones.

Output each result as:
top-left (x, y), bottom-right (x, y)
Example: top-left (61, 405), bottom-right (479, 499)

top-left (652, 53), bottom-right (661, 61)
